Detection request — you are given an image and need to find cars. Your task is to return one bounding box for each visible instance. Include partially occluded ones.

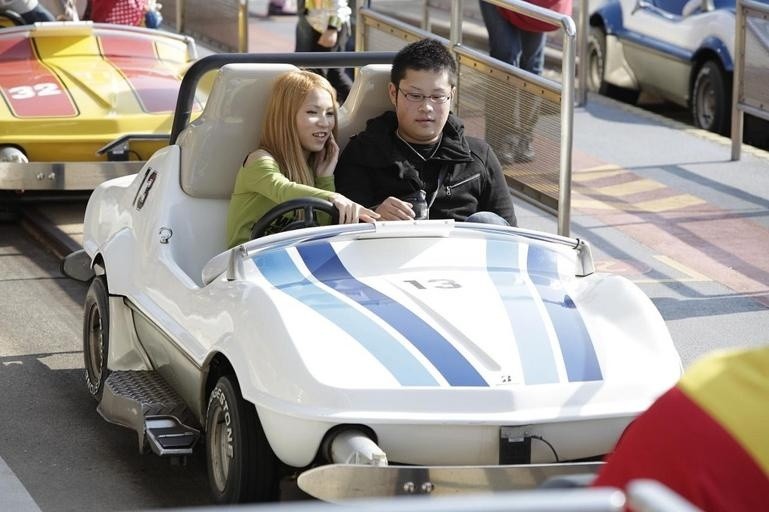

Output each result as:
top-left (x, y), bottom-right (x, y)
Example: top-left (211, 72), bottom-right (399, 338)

top-left (580, 0), bottom-right (769, 134)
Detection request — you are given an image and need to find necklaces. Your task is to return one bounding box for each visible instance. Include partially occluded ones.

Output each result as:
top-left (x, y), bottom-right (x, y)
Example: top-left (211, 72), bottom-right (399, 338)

top-left (395, 126), bottom-right (445, 163)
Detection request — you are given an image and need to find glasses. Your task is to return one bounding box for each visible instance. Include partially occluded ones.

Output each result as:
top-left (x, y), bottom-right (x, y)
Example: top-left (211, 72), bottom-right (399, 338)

top-left (396, 87), bottom-right (452, 105)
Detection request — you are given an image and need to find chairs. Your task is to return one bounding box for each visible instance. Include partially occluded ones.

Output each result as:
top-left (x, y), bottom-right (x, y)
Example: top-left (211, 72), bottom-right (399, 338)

top-left (173, 64), bottom-right (396, 288)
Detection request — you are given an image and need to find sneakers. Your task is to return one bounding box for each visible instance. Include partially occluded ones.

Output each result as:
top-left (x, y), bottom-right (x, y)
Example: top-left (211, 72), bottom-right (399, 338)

top-left (491, 133), bottom-right (535, 166)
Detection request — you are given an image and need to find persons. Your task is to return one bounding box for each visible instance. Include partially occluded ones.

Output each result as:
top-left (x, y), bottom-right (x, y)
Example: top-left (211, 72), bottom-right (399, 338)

top-left (292, 1), bottom-right (356, 105)
top-left (332, 38), bottom-right (518, 230)
top-left (477, 2), bottom-right (547, 166)
top-left (223, 68), bottom-right (381, 254)
